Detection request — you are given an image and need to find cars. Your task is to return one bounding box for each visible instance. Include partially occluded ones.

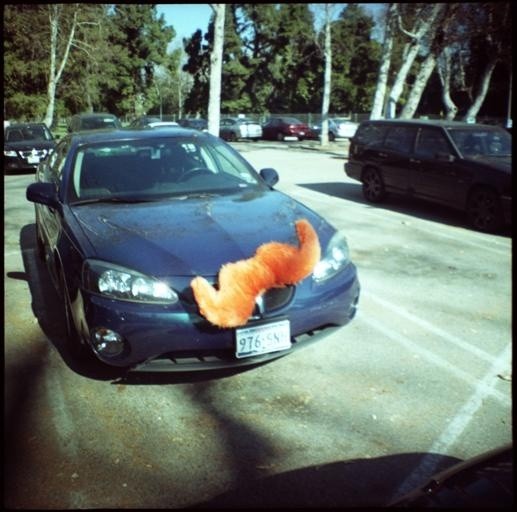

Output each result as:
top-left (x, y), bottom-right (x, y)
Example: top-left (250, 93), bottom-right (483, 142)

top-left (142, 116), bottom-right (320, 141)
top-left (328, 117), bottom-right (360, 141)
top-left (4, 122), bottom-right (57, 173)
top-left (67, 113), bottom-right (122, 133)
top-left (26, 127), bottom-right (361, 378)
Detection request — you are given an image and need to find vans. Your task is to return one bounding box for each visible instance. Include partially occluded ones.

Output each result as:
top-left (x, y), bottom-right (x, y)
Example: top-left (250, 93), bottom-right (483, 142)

top-left (344, 120), bottom-right (512, 231)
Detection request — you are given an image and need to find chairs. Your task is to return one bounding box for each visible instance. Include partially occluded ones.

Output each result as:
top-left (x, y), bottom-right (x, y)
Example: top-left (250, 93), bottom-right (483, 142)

top-left (160, 145), bottom-right (192, 183)
